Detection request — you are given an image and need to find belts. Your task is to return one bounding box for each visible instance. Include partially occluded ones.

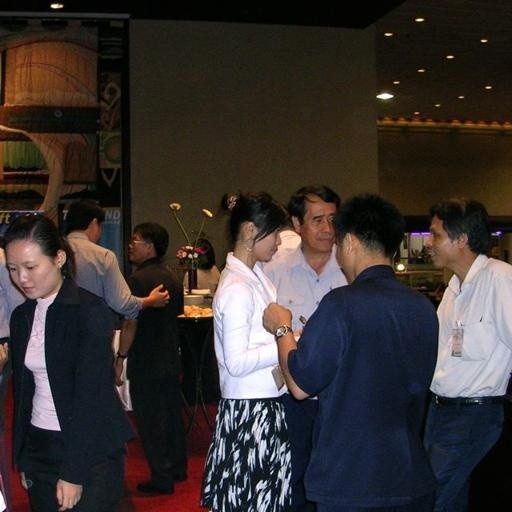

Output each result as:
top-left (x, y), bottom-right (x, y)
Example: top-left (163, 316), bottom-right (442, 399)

top-left (434, 394), bottom-right (508, 406)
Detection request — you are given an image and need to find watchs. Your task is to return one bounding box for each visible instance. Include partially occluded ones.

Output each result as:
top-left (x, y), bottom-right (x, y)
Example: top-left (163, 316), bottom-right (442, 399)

top-left (116, 351), bottom-right (127, 359)
top-left (274, 323), bottom-right (294, 339)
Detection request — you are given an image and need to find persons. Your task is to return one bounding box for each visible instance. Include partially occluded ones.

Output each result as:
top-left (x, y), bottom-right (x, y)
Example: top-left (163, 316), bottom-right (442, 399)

top-left (262, 184), bottom-right (353, 512)
top-left (0, 244), bottom-right (28, 511)
top-left (263, 193), bottom-right (439, 511)
top-left (112, 222), bottom-right (187, 493)
top-left (182, 238), bottom-right (221, 289)
top-left (422, 197), bottom-right (512, 511)
top-left (3, 216), bottom-right (137, 512)
top-left (61, 203), bottom-right (170, 317)
top-left (199, 195), bottom-right (306, 512)
top-left (258, 202), bottom-right (302, 271)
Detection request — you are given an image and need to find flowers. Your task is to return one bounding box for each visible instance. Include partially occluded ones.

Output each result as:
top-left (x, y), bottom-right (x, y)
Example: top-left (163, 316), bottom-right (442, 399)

top-left (169, 203), bottom-right (215, 267)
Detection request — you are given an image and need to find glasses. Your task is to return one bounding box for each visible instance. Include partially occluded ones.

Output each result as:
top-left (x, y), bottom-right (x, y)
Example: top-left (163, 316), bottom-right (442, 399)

top-left (129, 239), bottom-right (147, 246)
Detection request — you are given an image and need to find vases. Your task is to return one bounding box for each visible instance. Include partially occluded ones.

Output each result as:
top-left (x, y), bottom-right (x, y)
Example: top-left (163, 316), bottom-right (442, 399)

top-left (189, 259), bottom-right (199, 296)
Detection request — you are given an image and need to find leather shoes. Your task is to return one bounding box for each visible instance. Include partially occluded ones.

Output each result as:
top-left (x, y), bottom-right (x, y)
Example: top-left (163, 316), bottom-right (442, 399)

top-left (175, 473), bottom-right (188, 482)
top-left (137, 480), bottom-right (175, 494)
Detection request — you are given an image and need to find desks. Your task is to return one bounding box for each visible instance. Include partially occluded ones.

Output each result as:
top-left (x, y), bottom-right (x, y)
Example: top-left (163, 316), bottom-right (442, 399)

top-left (170, 314), bottom-right (214, 436)
